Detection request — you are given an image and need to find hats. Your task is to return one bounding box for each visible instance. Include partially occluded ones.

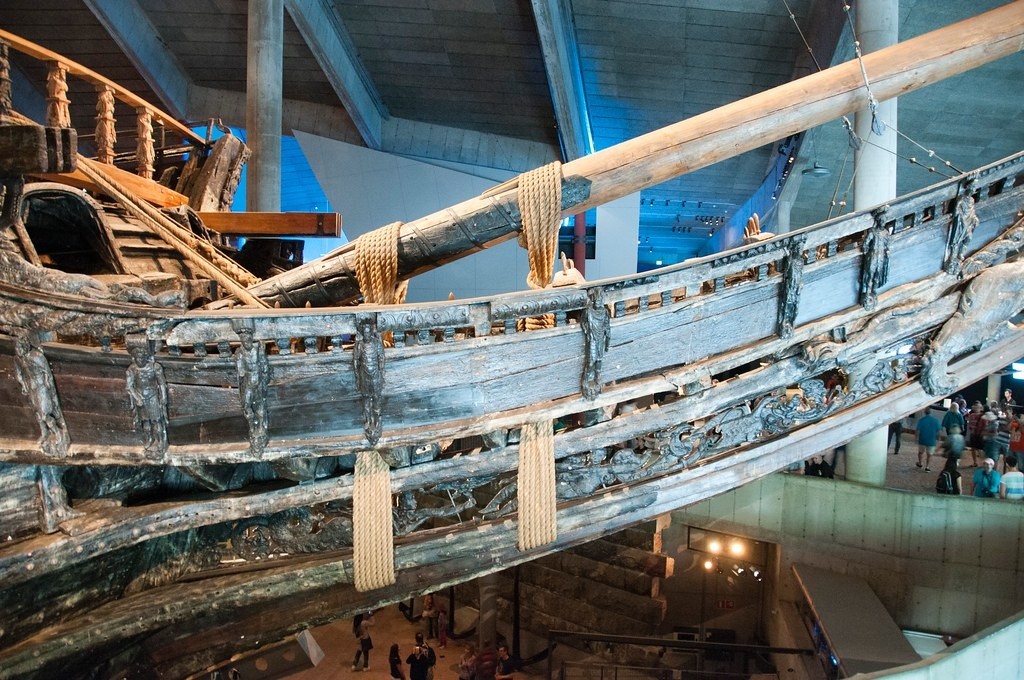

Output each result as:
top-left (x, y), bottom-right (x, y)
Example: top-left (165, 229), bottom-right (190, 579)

top-left (982, 458), bottom-right (994, 466)
top-left (981, 412), bottom-right (997, 421)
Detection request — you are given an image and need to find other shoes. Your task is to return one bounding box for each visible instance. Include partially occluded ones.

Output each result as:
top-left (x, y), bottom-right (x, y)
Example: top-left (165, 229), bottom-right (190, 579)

top-left (926, 468), bottom-right (931, 472)
top-left (916, 462), bottom-right (923, 468)
top-left (969, 464), bottom-right (977, 468)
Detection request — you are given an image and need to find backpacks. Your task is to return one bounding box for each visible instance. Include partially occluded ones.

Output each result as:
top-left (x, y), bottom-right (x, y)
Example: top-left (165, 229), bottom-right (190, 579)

top-left (936, 470), bottom-right (956, 495)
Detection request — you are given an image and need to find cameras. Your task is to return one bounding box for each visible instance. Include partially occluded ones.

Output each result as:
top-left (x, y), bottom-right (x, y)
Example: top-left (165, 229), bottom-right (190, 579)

top-left (368, 610), bottom-right (371, 615)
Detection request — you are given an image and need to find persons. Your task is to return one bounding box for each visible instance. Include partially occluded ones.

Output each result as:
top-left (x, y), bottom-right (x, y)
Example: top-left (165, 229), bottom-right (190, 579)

top-left (458, 644), bottom-right (477, 680)
top-left (999, 455), bottom-right (1024, 501)
top-left (889, 418), bottom-right (903, 456)
top-left (942, 389), bottom-right (1024, 501)
top-left (423, 590), bottom-right (448, 651)
top-left (936, 455), bottom-right (963, 496)
top-left (783, 452), bottom-right (834, 480)
top-left (389, 643), bottom-right (405, 680)
top-left (406, 632), bottom-right (437, 680)
top-left (494, 644), bottom-right (516, 680)
top-left (350, 609), bottom-right (376, 672)
top-left (914, 406), bottom-right (942, 473)
